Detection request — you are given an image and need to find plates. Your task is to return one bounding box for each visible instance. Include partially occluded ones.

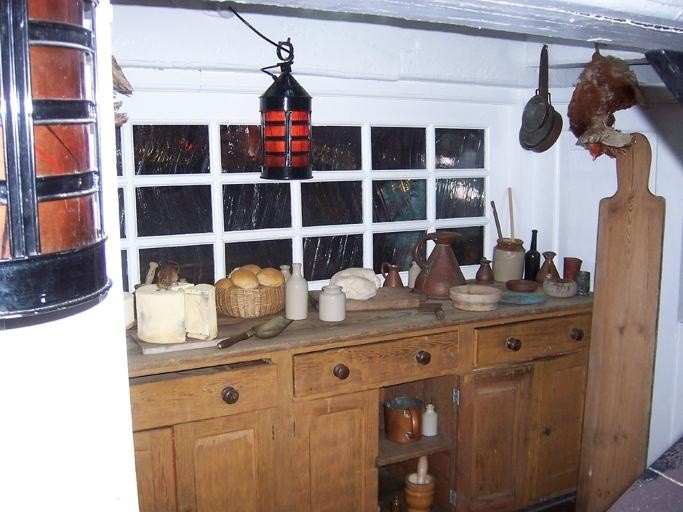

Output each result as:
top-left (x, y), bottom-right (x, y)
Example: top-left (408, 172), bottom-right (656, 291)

top-left (500, 290), bottom-right (546, 305)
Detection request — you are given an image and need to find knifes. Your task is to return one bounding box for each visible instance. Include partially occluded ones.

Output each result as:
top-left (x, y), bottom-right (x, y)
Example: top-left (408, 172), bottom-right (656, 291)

top-left (417, 303), bottom-right (446, 321)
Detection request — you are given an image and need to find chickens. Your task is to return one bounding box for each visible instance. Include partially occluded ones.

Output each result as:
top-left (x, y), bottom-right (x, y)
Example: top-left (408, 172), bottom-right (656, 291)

top-left (567, 44), bottom-right (648, 162)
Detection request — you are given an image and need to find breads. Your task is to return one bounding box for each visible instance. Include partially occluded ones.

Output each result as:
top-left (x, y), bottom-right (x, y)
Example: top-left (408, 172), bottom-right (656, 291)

top-left (214, 264), bottom-right (285, 289)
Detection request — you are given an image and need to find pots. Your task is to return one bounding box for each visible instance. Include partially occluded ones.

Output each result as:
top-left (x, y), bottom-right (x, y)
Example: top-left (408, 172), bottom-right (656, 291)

top-left (518, 46), bottom-right (564, 154)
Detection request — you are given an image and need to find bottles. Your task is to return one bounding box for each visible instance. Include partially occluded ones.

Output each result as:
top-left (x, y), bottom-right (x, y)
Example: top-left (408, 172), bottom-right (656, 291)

top-left (524, 229), bottom-right (541, 280)
top-left (422, 404), bottom-right (439, 438)
top-left (493, 238), bottom-right (527, 285)
top-left (285, 262), bottom-right (309, 321)
top-left (536, 250), bottom-right (563, 284)
top-left (318, 284), bottom-right (347, 323)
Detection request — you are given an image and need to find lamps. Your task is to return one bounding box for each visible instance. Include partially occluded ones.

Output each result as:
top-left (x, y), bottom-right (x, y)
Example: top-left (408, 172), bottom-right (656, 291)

top-left (227, 5), bottom-right (313, 180)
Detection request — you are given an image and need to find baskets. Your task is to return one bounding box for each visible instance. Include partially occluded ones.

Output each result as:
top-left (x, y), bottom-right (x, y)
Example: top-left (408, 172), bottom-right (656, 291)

top-left (216, 283), bottom-right (286, 319)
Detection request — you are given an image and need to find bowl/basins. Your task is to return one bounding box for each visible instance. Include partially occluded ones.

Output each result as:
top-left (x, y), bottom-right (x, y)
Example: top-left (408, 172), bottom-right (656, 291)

top-left (505, 279), bottom-right (539, 293)
top-left (449, 285), bottom-right (501, 312)
top-left (543, 278), bottom-right (578, 298)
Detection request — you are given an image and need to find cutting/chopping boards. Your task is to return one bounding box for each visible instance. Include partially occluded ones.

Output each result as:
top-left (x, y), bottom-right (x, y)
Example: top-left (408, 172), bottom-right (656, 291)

top-left (308, 285), bottom-right (420, 313)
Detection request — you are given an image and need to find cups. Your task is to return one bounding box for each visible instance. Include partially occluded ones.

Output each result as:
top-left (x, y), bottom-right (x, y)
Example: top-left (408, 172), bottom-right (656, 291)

top-left (562, 256), bottom-right (583, 281)
top-left (383, 396), bottom-right (424, 444)
top-left (576, 271), bottom-right (591, 296)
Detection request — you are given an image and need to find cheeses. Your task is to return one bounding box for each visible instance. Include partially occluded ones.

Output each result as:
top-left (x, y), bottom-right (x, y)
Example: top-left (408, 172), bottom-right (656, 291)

top-left (135, 282), bottom-right (217, 344)
